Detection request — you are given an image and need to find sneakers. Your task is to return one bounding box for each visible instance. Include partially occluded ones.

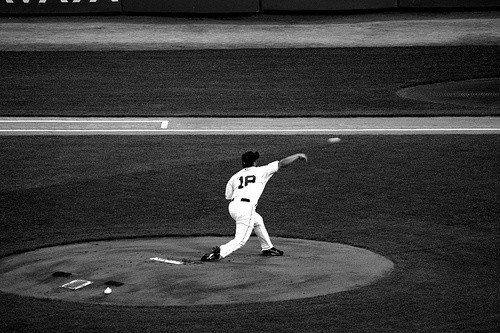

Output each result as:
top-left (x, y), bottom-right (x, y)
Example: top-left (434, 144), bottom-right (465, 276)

top-left (201, 246), bottom-right (220, 263)
top-left (261, 247), bottom-right (284, 257)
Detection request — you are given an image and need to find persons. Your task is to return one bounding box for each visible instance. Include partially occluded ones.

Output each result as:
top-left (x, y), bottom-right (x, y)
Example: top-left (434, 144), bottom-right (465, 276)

top-left (201, 151), bottom-right (308, 262)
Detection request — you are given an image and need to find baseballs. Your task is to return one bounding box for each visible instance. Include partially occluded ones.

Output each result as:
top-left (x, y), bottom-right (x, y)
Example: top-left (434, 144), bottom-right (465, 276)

top-left (327, 137), bottom-right (341, 144)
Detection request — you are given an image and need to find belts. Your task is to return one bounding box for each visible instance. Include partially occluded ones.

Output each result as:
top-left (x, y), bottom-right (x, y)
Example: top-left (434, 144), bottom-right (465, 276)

top-left (230, 198), bottom-right (250, 202)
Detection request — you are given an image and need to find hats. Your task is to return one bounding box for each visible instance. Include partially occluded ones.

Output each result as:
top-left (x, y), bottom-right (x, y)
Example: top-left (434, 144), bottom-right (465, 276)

top-left (242, 150), bottom-right (260, 163)
